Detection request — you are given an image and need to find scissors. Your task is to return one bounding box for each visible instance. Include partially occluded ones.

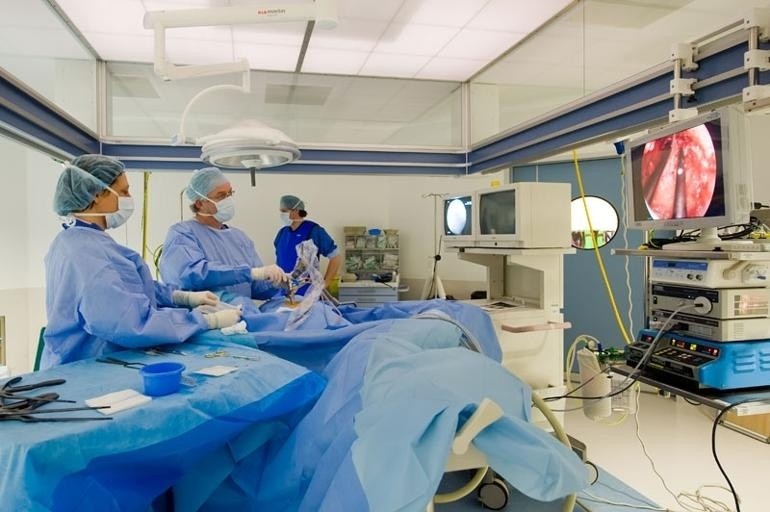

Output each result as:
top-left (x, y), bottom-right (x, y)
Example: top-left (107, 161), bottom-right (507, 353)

top-left (204, 351), bottom-right (257, 359)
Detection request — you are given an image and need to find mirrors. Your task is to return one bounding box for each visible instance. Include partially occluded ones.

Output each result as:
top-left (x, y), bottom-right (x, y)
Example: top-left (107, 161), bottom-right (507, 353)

top-left (570, 193), bottom-right (620, 251)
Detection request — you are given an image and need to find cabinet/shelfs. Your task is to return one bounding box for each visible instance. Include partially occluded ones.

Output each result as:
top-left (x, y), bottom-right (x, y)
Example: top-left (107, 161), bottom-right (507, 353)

top-left (337, 245), bottom-right (409, 310)
top-left (441, 245), bottom-right (580, 440)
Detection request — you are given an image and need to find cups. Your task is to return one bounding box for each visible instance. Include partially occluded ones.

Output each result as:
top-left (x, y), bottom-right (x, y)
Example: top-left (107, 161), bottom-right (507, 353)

top-left (575, 348), bottom-right (613, 421)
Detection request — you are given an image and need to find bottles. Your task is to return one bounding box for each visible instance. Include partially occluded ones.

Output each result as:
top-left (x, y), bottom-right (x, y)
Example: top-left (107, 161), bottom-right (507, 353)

top-left (384, 229), bottom-right (400, 249)
top-left (345, 229), bottom-right (381, 251)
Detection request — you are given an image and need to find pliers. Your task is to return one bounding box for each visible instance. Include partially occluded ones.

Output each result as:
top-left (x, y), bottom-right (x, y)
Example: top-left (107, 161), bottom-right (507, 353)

top-left (139, 347), bottom-right (187, 356)
top-left (95, 357), bottom-right (146, 369)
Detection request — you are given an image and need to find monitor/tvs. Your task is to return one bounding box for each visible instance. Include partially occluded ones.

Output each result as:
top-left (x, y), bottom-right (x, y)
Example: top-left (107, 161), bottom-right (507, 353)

top-left (440, 192), bottom-right (474, 248)
top-left (626, 105), bottom-right (753, 252)
top-left (474, 182), bottom-right (571, 249)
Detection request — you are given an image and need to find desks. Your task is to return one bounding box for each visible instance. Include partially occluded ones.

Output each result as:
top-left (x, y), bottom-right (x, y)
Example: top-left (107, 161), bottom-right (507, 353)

top-left (2, 340), bottom-right (329, 512)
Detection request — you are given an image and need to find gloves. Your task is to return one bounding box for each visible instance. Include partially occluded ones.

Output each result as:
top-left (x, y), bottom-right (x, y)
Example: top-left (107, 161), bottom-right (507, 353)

top-left (173, 290), bottom-right (220, 307)
top-left (273, 273), bottom-right (293, 288)
top-left (207, 308), bottom-right (243, 330)
top-left (251, 263), bottom-right (287, 286)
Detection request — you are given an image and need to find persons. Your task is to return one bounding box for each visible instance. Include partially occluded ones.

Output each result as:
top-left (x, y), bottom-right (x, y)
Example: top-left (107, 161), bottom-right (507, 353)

top-left (158, 168), bottom-right (305, 306)
top-left (38, 154), bottom-right (243, 372)
top-left (272, 196), bottom-right (343, 298)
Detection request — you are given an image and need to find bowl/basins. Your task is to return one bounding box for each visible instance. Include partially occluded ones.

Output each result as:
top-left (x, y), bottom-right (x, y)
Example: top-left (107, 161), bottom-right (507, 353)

top-left (139, 361), bottom-right (186, 397)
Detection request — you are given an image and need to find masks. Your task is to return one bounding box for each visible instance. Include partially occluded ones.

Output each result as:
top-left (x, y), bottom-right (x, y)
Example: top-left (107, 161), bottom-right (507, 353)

top-left (198, 193), bottom-right (234, 223)
top-left (97, 179), bottom-right (134, 230)
top-left (279, 207), bottom-right (297, 226)
